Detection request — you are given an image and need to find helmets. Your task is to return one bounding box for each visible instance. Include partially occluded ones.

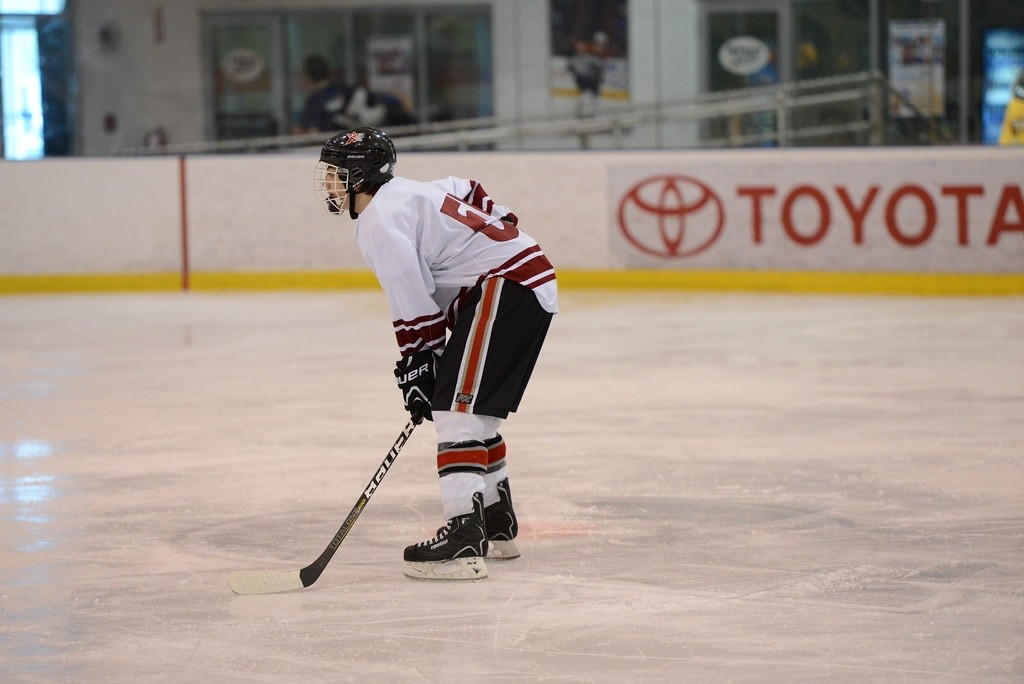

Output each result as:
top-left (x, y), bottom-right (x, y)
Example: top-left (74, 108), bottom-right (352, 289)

top-left (320, 127), bottom-right (397, 214)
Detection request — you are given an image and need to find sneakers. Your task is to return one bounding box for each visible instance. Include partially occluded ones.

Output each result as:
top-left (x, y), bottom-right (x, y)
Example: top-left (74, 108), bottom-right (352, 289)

top-left (403, 492), bottom-right (489, 579)
top-left (483, 477), bottom-right (520, 559)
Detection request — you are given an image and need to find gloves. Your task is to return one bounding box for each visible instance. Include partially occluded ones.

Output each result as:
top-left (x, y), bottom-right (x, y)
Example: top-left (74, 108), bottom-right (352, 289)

top-left (394, 350), bottom-right (440, 425)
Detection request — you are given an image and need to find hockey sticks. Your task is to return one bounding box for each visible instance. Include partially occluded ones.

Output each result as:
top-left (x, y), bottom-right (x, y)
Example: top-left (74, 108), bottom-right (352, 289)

top-left (227, 408), bottom-right (424, 595)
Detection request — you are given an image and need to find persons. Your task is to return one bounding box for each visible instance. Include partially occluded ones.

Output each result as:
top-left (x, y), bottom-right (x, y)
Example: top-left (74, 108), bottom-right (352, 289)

top-left (314, 128), bottom-right (559, 578)
top-left (297, 54), bottom-right (419, 139)
top-left (566, 31), bottom-right (611, 118)
